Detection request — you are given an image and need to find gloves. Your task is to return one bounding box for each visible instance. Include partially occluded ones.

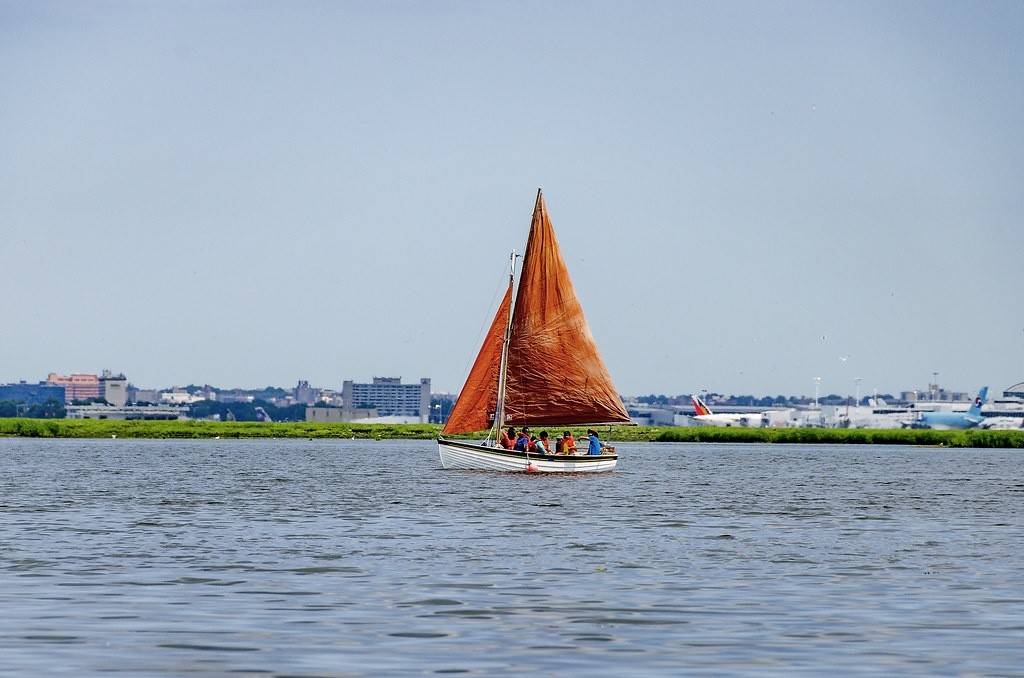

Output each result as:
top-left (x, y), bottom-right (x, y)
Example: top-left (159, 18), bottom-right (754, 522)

top-left (531, 437), bottom-right (537, 441)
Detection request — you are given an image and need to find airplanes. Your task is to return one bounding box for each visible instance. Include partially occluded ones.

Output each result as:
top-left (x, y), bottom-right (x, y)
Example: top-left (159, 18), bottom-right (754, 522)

top-left (689, 395), bottom-right (802, 429)
top-left (901, 384), bottom-right (995, 430)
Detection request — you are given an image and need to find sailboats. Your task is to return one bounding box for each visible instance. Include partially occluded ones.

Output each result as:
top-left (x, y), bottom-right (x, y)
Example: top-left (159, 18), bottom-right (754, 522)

top-left (434, 186), bottom-right (641, 474)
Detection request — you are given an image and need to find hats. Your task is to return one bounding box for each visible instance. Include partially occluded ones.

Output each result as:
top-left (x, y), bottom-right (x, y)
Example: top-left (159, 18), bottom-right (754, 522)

top-left (556, 435), bottom-right (563, 439)
top-left (587, 429), bottom-right (598, 438)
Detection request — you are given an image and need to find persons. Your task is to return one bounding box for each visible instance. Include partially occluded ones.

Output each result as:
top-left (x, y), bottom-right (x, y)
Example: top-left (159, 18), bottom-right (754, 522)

top-left (499, 426), bottom-right (602, 456)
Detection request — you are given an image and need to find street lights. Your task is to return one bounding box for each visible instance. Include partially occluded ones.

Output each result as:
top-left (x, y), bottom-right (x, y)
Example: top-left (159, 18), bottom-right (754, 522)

top-left (813, 377), bottom-right (822, 407)
top-left (855, 378), bottom-right (861, 407)
